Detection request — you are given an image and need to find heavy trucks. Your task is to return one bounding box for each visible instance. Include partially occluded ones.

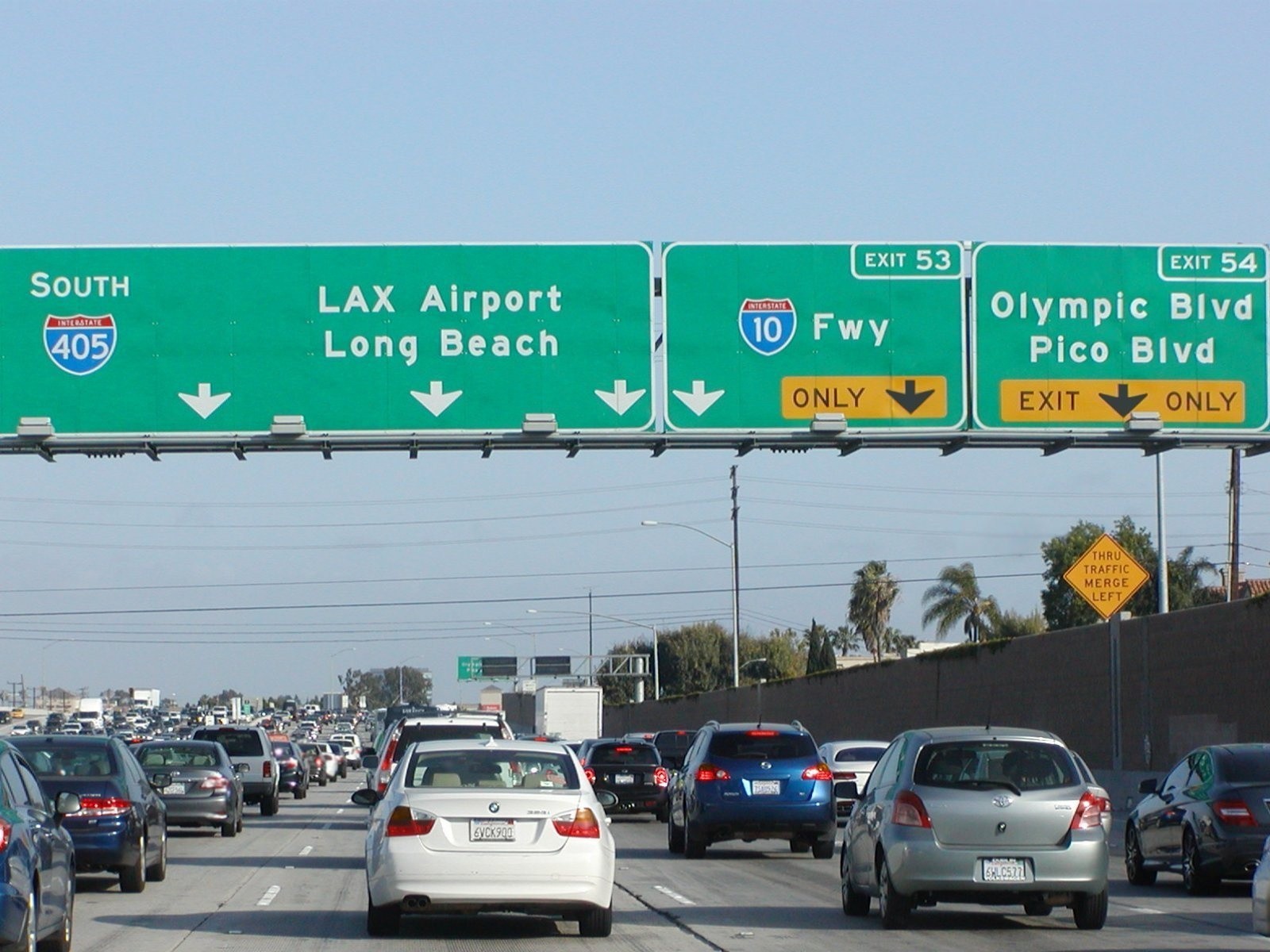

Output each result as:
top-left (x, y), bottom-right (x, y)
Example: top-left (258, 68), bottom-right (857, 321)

top-left (75, 698), bottom-right (104, 733)
top-left (323, 694), bottom-right (349, 714)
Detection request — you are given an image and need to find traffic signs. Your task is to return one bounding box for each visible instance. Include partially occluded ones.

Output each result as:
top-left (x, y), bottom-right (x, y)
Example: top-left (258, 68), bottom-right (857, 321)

top-left (0, 243), bottom-right (1265, 439)
top-left (456, 656), bottom-right (508, 682)
top-left (1066, 533), bottom-right (1150, 622)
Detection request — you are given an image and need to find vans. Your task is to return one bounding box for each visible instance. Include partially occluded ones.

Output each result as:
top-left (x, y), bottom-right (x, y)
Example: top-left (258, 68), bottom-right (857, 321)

top-left (329, 733), bottom-right (363, 769)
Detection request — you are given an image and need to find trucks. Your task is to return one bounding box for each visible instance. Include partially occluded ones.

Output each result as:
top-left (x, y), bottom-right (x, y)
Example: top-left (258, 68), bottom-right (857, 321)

top-left (535, 686), bottom-right (603, 742)
top-left (500, 692), bottom-right (540, 735)
top-left (131, 688), bottom-right (161, 712)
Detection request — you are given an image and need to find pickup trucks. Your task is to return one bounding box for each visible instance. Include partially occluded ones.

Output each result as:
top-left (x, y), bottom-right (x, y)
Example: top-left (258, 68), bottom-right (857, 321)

top-left (650, 730), bottom-right (702, 770)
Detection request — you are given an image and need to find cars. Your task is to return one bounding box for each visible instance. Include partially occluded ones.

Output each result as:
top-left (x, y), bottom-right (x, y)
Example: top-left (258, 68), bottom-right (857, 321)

top-left (1124, 743), bottom-right (1270, 896)
top-left (517, 733), bottom-right (561, 775)
top-left (292, 744), bottom-right (328, 785)
top-left (257, 740), bottom-right (309, 799)
top-left (130, 741), bottom-right (250, 834)
top-left (307, 742), bottom-right (338, 782)
top-left (0, 737), bottom-right (84, 951)
top-left (833, 724), bottom-right (1110, 929)
top-left (350, 737), bottom-right (620, 935)
top-left (573, 737), bottom-right (647, 768)
top-left (329, 743), bottom-right (347, 778)
top-left (2, 735), bottom-right (173, 892)
top-left (0, 701), bottom-right (456, 747)
top-left (585, 741), bottom-right (670, 822)
top-left (952, 749), bottom-right (1112, 838)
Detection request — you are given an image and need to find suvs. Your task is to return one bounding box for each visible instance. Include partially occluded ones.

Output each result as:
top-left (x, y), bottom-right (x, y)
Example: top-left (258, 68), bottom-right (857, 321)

top-left (188, 725), bottom-right (280, 814)
top-left (325, 740), bottom-right (358, 770)
top-left (363, 716), bottom-right (526, 819)
top-left (812, 739), bottom-right (916, 826)
top-left (666, 717), bottom-right (837, 862)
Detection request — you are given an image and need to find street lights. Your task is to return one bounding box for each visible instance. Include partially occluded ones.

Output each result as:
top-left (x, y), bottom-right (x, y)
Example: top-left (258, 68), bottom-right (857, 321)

top-left (641, 519), bottom-right (740, 689)
top-left (481, 608), bottom-right (662, 700)
